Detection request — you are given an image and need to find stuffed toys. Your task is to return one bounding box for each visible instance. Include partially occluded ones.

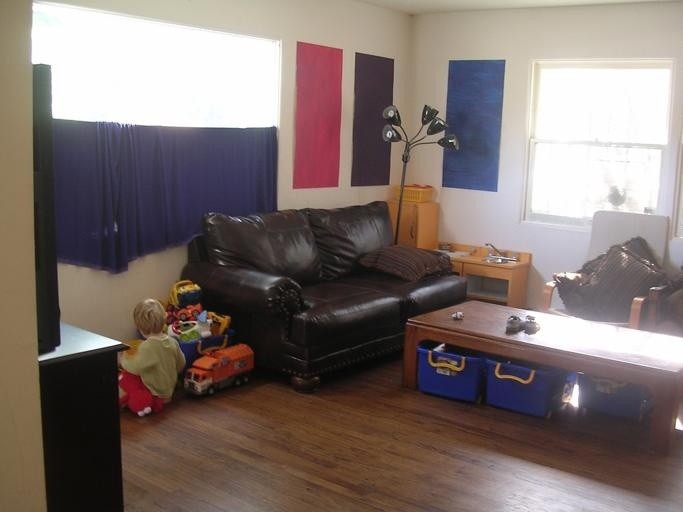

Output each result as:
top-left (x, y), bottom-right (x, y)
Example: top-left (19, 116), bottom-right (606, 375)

top-left (117, 370), bottom-right (165, 418)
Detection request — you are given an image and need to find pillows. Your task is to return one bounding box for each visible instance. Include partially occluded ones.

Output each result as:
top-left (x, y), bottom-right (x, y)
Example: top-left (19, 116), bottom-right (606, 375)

top-left (550, 235), bottom-right (665, 319)
top-left (361, 243), bottom-right (456, 284)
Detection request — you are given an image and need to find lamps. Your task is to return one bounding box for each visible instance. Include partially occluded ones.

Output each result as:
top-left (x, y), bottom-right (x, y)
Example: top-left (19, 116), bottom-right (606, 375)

top-left (377, 102), bottom-right (461, 249)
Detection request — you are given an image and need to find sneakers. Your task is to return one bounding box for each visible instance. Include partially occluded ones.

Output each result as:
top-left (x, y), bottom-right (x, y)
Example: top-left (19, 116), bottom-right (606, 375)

top-left (506, 315), bottom-right (526, 333)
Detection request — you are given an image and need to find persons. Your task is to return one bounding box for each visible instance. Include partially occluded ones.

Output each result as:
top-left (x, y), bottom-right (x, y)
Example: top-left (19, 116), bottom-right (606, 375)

top-left (116, 297), bottom-right (187, 415)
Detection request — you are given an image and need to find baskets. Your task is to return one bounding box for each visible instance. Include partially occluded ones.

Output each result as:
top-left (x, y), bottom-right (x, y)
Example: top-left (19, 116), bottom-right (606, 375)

top-left (396, 185), bottom-right (433, 201)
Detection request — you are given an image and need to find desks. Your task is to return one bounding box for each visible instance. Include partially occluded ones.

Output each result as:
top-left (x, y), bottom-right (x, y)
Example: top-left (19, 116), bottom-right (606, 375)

top-left (37, 324), bottom-right (131, 512)
top-left (440, 243), bottom-right (532, 310)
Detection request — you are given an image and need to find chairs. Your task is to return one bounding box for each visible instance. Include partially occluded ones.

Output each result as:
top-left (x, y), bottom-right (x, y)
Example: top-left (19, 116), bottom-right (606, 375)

top-left (542, 208), bottom-right (670, 327)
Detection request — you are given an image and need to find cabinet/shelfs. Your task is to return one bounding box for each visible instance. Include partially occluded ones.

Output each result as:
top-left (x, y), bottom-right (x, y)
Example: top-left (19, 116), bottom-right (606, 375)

top-left (383, 197), bottom-right (440, 250)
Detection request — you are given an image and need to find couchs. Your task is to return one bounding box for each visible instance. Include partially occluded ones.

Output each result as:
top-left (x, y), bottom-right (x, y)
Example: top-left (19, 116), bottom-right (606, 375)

top-left (178, 199), bottom-right (472, 392)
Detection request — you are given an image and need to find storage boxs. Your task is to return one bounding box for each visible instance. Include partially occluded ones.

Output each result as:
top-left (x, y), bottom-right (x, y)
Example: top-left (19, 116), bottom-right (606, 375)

top-left (481, 354), bottom-right (580, 421)
top-left (573, 369), bottom-right (656, 425)
top-left (414, 335), bottom-right (486, 407)
